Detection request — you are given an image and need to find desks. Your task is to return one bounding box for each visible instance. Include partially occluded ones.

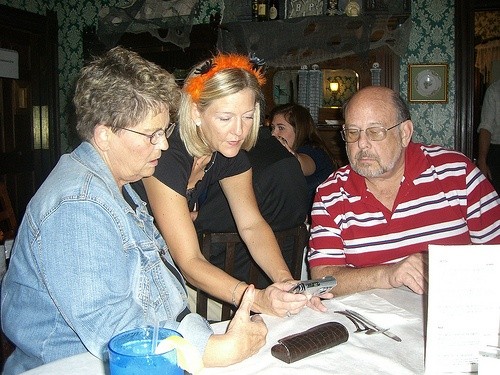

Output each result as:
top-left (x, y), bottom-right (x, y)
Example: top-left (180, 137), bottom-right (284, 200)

top-left (17, 286), bottom-right (426, 375)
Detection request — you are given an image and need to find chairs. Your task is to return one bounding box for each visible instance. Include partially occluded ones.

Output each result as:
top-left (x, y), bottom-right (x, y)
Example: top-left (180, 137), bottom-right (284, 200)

top-left (195, 224), bottom-right (305, 324)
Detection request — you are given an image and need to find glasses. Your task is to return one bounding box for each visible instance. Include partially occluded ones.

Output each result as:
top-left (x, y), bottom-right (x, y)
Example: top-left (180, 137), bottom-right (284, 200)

top-left (341, 118), bottom-right (411, 143)
top-left (104, 121), bottom-right (176, 145)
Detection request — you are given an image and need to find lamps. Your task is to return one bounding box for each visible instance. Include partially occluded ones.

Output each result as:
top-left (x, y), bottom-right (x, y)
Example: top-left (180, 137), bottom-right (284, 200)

top-left (330, 82), bottom-right (339, 106)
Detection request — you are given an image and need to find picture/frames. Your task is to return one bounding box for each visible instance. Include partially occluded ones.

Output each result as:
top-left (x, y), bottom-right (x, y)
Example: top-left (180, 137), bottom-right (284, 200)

top-left (407, 63), bottom-right (448, 103)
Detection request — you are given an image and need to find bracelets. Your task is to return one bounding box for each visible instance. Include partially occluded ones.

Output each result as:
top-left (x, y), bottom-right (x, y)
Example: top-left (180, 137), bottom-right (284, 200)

top-left (232, 281), bottom-right (246, 309)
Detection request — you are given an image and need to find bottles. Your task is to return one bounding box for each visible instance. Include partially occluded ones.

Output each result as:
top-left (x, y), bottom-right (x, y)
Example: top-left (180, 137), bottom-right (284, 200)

top-left (257, 0), bottom-right (269, 21)
top-left (269, 0), bottom-right (279, 21)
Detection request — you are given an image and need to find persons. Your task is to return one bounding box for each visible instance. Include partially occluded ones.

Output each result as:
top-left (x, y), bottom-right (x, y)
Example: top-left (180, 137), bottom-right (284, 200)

top-left (477, 78), bottom-right (500, 197)
top-left (188, 102), bottom-right (336, 324)
top-left (307, 86), bottom-right (500, 295)
top-left (122, 53), bottom-right (333, 318)
top-left (0, 45), bottom-right (268, 375)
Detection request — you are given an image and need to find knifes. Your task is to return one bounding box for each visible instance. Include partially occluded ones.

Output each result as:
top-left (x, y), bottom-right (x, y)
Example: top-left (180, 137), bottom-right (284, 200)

top-left (347, 310), bottom-right (401, 342)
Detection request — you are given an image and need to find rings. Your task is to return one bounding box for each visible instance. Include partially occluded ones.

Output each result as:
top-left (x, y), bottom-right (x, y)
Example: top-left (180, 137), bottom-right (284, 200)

top-left (287, 312), bottom-right (291, 317)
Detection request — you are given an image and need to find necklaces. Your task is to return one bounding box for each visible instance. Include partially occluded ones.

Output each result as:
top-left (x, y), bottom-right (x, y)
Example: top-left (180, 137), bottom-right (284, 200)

top-left (194, 151), bottom-right (217, 172)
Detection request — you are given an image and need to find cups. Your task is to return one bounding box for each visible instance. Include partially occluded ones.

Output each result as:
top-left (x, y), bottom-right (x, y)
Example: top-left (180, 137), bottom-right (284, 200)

top-left (108, 326), bottom-right (184, 375)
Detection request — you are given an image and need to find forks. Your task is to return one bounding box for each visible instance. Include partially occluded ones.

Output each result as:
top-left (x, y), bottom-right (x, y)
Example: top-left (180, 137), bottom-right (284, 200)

top-left (334, 311), bottom-right (390, 334)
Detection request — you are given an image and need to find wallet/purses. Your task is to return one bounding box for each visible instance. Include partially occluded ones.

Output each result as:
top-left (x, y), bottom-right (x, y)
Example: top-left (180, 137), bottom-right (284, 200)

top-left (271, 321), bottom-right (348, 364)
